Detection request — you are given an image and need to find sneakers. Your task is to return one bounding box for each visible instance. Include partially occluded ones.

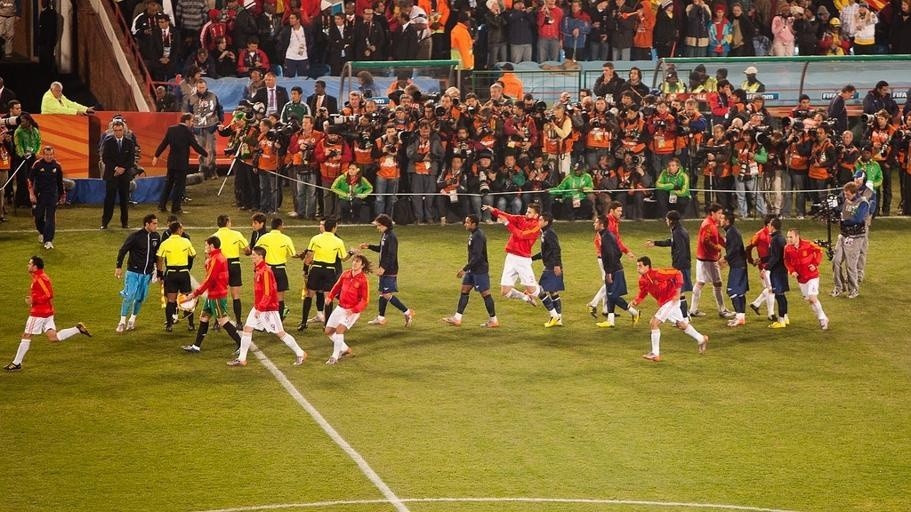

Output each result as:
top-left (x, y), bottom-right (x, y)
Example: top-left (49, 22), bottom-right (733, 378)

top-left (43, 241), bottom-right (53, 250)
top-left (642, 353), bottom-right (660, 362)
top-left (442, 318), bottom-right (460, 327)
top-left (2, 360), bottom-right (21, 371)
top-left (698, 335), bottom-right (708, 354)
top-left (38, 234), bottom-right (43, 242)
top-left (479, 321), bottom-right (498, 328)
top-left (75, 322), bottom-right (91, 337)
top-left (115, 308), bottom-right (415, 365)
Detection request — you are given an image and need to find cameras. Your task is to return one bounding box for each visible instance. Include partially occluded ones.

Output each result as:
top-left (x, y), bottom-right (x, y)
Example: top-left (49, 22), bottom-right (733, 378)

top-left (327, 148), bottom-right (341, 158)
top-left (383, 141), bottom-right (398, 154)
top-left (631, 153), bottom-right (646, 166)
top-left (449, 175), bottom-right (458, 186)
top-left (736, 160), bottom-right (748, 182)
top-left (301, 142), bottom-right (314, 162)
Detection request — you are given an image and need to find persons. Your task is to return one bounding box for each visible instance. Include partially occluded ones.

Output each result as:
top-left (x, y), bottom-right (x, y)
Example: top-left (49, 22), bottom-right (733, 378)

top-left (115, 214), bottom-right (196, 332)
top-left (132, 0), bottom-right (910, 231)
top-left (593, 214), bottom-right (641, 327)
top-left (782, 227), bottom-right (830, 331)
top-left (645, 210), bottom-right (694, 328)
top-left (211, 215), bottom-right (251, 331)
top-left (586, 201), bottom-right (635, 319)
top-left (152, 114), bottom-right (209, 214)
top-left (360, 213), bottom-right (416, 328)
top-left (2, 255), bottom-right (92, 371)
top-left (226, 248), bottom-right (308, 367)
top-left (532, 210), bottom-right (565, 328)
top-left (746, 213), bottom-right (790, 328)
top-left (298, 217), bottom-right (359, 330)
top-left (1, 2), bottom-right (145, 229)
top-left (830, 181), bottom-right (869, 298)
top-left (483, 202), bottom-right (542, 308)
top-left (27, 146), bottom-right (67, 250)
top-left (245, 211), bottom-right (303, 322)
top-left (324, 254), bottom-right (374, 366)
top-left (442, 215), bottom-right (501, 328)
top-left (719, 211), bottom-right (749, 328)
top-left (689, 202), bottom-right (736, 319)
top-left (182, 235), bottom-right (242, 354)
top-left (627, 256), bottom-right (709, 362)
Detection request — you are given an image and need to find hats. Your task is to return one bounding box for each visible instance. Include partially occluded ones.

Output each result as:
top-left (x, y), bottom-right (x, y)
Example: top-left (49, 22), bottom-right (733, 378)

top-left (743, 67), bottom-right (757, 74)
top-left (852, 170), bottom-right (867, 177)
